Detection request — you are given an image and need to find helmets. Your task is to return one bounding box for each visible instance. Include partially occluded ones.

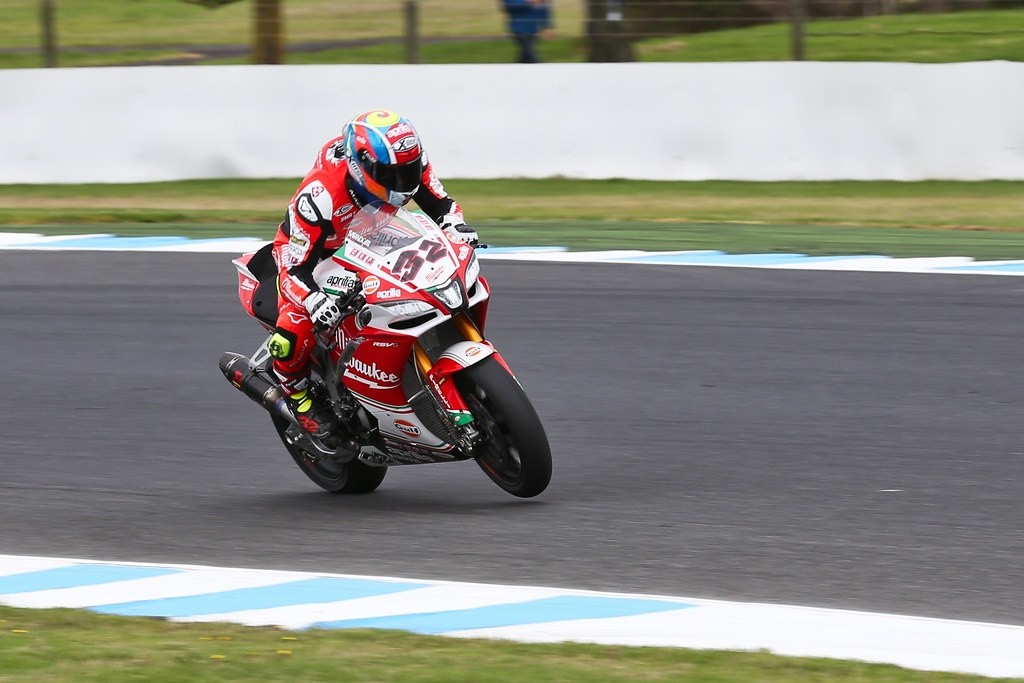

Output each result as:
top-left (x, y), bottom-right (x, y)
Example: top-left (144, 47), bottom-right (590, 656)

top-left (341, 110), bottom-right (424, 209)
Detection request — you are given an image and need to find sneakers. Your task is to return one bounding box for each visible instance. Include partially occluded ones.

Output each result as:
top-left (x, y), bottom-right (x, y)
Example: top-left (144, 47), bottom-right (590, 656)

top-left (286, 391), bottom-right (335, 440)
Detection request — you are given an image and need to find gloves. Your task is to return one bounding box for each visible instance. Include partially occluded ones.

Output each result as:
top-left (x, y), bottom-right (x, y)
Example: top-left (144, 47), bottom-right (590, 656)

top-left (302, 288), bottom-right (342, 331)
top-left (435, 213), bottom-right (478, 248)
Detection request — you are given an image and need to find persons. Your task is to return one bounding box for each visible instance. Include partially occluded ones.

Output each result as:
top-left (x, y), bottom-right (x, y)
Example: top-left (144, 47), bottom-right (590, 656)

top-left (267, 110), bottom-right (478, 439)
top-left (503, 0), bottom-right (554, 63)
top-left (586, 0), bottom-right (632, 62)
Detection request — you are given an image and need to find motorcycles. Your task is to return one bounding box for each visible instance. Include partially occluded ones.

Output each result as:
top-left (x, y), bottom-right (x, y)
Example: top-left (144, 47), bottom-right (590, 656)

top-left (212, 211), bottom-right (554, 500)
